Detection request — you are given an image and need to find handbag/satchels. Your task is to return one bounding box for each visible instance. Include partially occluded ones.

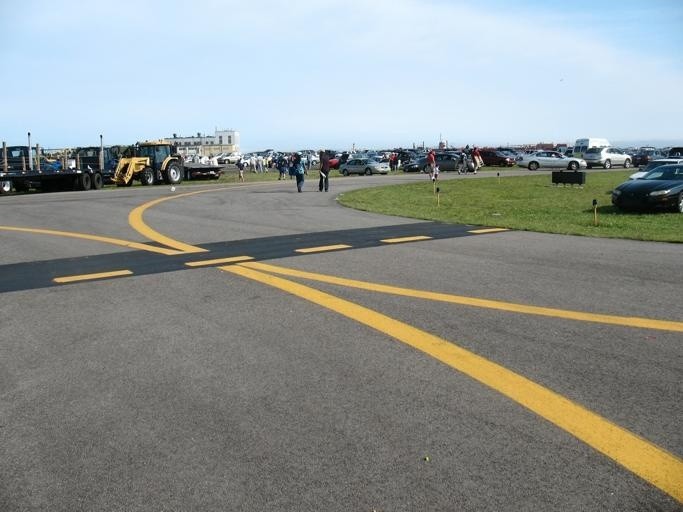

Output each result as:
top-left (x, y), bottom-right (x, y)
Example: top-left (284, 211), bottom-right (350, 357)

top-left (289, 164), bottom-right (304, 176)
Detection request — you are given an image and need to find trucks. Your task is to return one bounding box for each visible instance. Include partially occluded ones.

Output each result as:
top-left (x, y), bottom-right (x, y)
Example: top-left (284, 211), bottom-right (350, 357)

top-left (0, 132), bottom-right (225, 196)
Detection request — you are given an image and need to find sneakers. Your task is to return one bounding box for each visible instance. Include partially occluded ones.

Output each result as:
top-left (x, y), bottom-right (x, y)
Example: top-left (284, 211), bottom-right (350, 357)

top-left (298, 187), bottom-right (329, 192)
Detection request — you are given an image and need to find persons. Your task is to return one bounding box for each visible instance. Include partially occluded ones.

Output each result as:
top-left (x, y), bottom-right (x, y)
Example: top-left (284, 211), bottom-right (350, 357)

top-left (193, 153), bottom-right (218, 175)
top-left (455, 147), bottom-right (483, 174)
top-left (427, 151), bottom-right (440, 180)
top-left (43, 153), bottom-right (58, 170)
top-left (237, 152), bottom-right (312, 180)
top-left (292, 152), bottom-right (308, 193)
top-left (390, 153), bottom-right (398, 171)
top-left (319, 152), bottom-right (330, 193)
